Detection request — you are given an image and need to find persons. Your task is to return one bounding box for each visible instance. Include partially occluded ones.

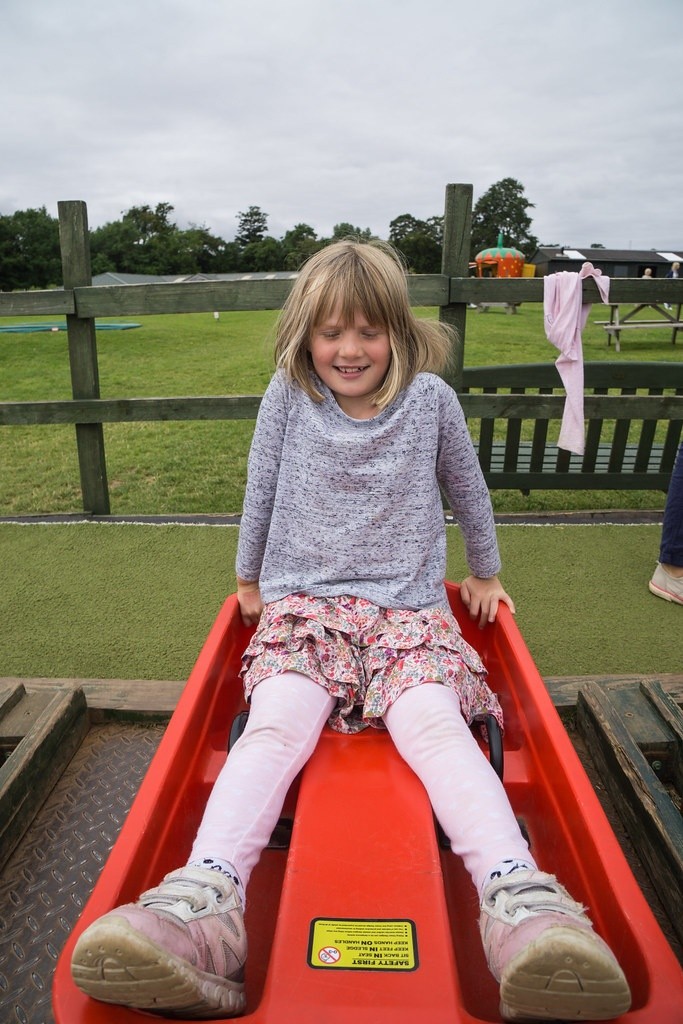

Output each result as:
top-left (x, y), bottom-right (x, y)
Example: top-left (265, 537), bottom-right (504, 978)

top-left (641, 262), bottom-right (681, 309)
top-left (72, 240), bottom-right (629, 1023)
top-left (650, 439), bottom-right (683, 604)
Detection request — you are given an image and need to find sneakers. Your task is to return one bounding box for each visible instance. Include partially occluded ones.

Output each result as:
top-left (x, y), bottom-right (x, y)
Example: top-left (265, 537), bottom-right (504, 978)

top-left (68, 865), bottom-right (245, 1014)
top-left (479, 869), bottom-right (632, 1021)
top-left (649, 560), bottom-right (682, 607)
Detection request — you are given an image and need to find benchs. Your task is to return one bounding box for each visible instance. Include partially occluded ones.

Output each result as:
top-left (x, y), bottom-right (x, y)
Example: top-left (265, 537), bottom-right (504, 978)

top-left (593, 319), bottom-right (683, 352)
top-left (464, 362), bottom-right (683, 496)
